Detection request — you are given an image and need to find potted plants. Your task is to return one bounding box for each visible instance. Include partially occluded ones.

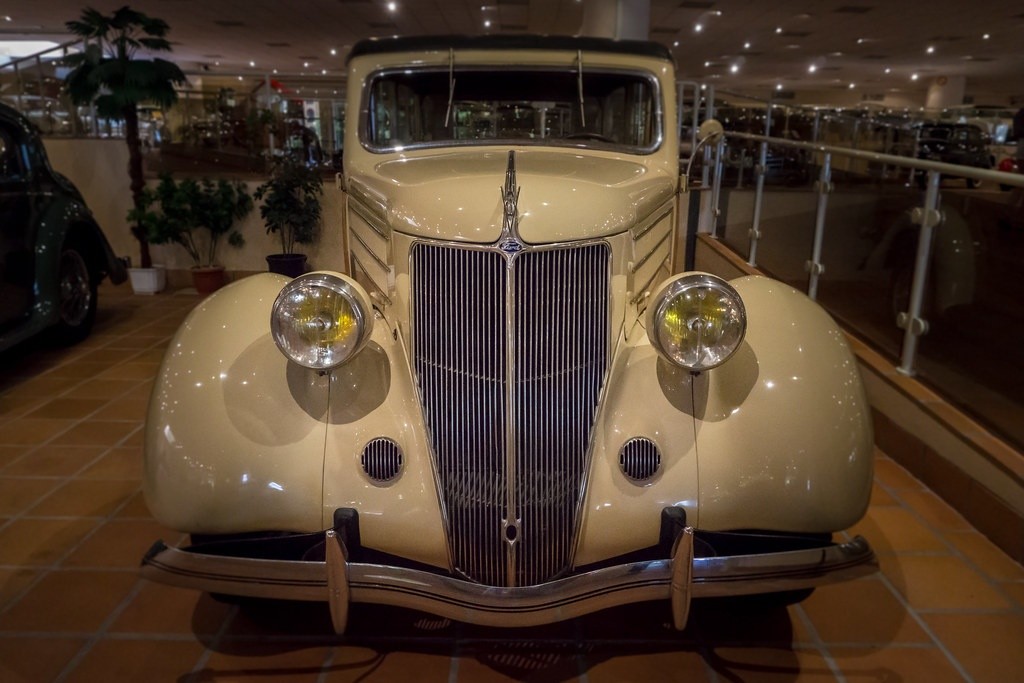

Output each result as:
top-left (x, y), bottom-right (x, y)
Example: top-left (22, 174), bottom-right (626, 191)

top-left (64, 4), bottom-right (192, 296)
top-left (124, 172), bottom-right (254, 299)
top-left (254, 148), bottom-right (326, 279)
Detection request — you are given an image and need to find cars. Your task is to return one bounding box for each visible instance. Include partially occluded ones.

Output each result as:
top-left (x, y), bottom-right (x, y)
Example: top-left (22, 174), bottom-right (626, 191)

top-left (143, 33), bottom-right (871, 631)
top-left (704, 99), bottom-right (1023, 196)
top-left (0, 102), bottom-right (122, 362)
